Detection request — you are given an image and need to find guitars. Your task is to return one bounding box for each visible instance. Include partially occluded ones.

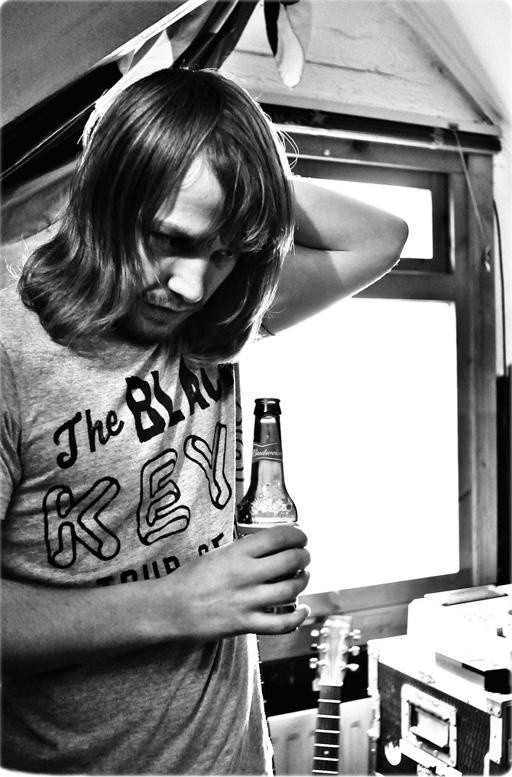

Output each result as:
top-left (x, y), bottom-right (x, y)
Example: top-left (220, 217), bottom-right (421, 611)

top-left (309, 615), bottom-right (362, 776)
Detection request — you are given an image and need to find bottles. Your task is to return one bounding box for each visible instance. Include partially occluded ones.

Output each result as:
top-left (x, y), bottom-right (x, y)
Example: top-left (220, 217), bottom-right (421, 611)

top-left (235, 398), bottom-right (299, 615)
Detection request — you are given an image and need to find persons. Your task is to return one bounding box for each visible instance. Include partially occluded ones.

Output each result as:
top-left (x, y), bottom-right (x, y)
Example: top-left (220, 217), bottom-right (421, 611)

top-left (1, 69), bottom-right (410, 775)
